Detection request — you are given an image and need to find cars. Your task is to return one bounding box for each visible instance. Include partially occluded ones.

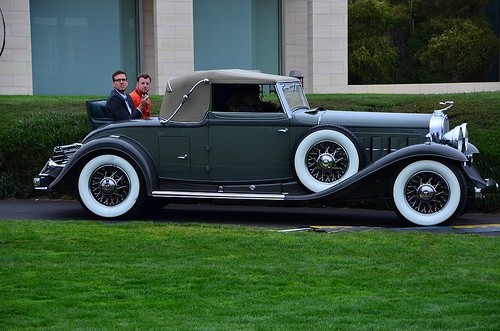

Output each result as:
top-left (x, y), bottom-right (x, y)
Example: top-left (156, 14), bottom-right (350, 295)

top-left (31, 69), bottom-right (498, 228)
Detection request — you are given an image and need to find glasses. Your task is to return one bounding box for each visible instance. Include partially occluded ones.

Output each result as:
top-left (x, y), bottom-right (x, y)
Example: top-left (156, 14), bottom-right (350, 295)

top-left (113, 78), bottom-right (127, 82)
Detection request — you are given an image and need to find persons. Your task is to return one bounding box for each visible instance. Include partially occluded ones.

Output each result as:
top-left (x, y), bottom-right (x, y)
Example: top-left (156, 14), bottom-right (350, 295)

top-left (129, 73), bottom-right (152, 119)
top-left (105, 71), bottom-right (150, 122)
